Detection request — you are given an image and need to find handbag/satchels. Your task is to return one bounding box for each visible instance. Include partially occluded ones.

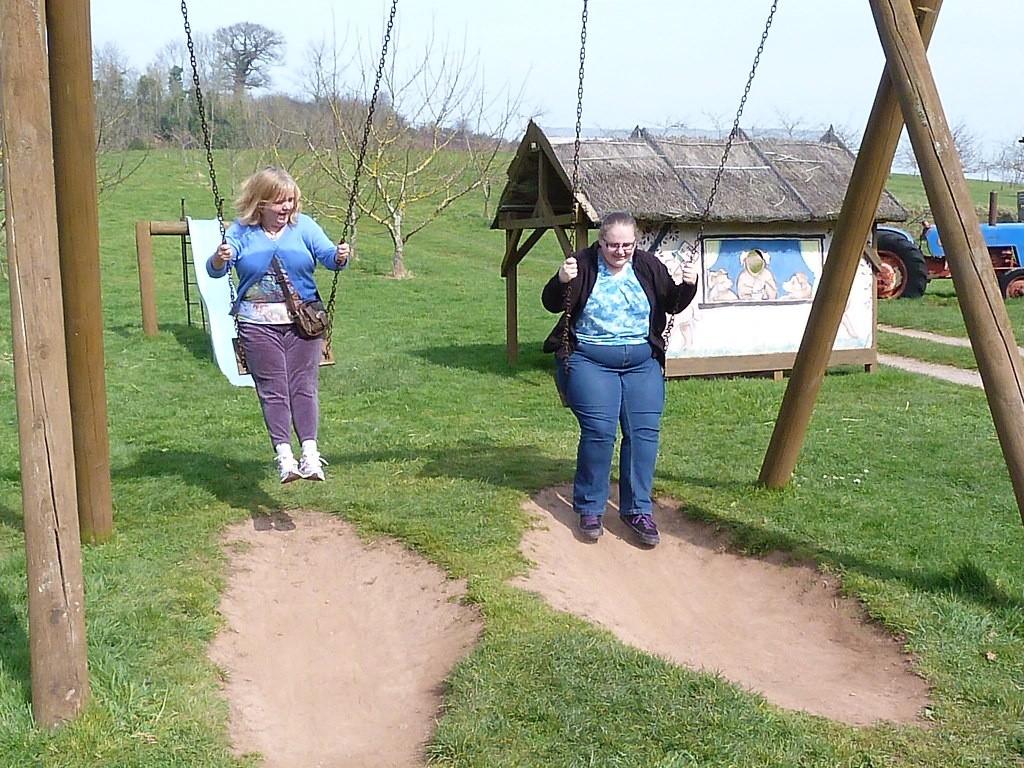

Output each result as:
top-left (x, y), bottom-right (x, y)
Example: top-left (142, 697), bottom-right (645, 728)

top-left (293, 298), bottom-right (332, 340)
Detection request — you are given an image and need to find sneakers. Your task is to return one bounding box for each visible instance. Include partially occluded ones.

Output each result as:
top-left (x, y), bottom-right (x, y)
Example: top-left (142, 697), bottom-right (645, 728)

top-left (578, 514), bottom-right (604, 538)
top-left (299, 448), bottom-right (330, 481)
top-left (619, 512), bottom-right (659, 546)
top-left (273, 452), bottom-right (302, 484)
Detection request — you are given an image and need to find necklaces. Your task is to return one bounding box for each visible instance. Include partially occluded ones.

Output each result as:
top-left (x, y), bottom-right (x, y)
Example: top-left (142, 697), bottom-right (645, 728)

top-left (261, 221), bottom-right (286, 235)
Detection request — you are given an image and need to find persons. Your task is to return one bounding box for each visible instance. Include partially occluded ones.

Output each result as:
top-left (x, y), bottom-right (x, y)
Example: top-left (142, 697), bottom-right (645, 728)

top-left (539, 212), bottom-right (698, 545)
top-left (205, 165), bottom-right (352, 485)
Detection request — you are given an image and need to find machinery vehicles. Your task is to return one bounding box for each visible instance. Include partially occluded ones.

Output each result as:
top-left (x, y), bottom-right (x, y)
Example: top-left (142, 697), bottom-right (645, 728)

top-left (876, 221), bottom-right (1024, 305)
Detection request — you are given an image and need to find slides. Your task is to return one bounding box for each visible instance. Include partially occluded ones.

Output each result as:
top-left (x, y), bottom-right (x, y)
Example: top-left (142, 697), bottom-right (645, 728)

top-left (185, 217), bottom-right (258, 389)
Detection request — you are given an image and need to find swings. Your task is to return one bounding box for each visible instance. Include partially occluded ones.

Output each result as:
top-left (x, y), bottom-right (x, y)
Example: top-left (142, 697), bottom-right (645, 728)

top-left (181, 0), bottom-right (400, 374)
top-left (552, 0), bottom-right (778, 409)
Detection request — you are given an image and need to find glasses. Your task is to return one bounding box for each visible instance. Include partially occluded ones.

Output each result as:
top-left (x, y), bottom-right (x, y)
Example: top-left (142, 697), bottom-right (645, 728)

top-left (600, 234), bottom-right (636, 250)
